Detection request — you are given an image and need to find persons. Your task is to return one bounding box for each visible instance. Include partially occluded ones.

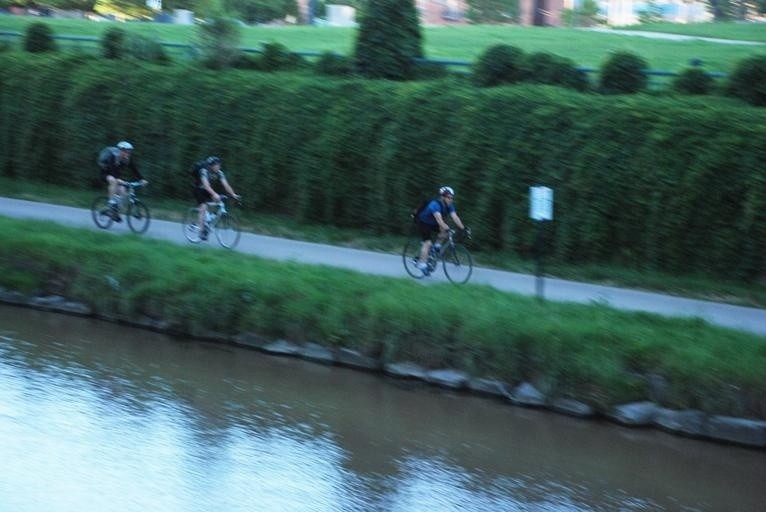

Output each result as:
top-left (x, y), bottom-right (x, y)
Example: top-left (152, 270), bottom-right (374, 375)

top-left (189, 155), bottom-right (242, 242)
top-left (415, 186), bottom-right (465, 270)
top-left (90, 141), bottom-right (148, 223)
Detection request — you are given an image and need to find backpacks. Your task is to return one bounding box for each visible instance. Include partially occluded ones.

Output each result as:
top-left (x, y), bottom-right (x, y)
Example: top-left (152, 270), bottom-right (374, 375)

top-left (410, 199), bottom-right (442, 221)
top-left (189, 161), bottom-right (208, 184)
top-left (93, 147), bottom-right (121, 177)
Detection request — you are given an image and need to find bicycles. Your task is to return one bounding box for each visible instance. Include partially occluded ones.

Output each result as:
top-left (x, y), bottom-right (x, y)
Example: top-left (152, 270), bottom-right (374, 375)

top-left (90, 180), bottom-right (151, 235)
top-left (402, 228), bottom-right (472, 284)
top-left (182, 195), bottom-right (241, 248)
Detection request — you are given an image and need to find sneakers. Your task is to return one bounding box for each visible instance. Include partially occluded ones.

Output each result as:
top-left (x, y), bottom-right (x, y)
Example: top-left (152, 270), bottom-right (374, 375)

top-left (106, 200), bottom-right (121, 212)
top-left (416, 263), bottom-right (429, 277)
top-left (431, 243), bottom-right (444, 258)
top-left (199, 232), bottom-right (207, 240)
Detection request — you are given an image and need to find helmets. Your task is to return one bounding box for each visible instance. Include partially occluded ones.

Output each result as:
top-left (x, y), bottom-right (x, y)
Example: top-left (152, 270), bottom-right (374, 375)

top-left (117, 141), bottom-right (134, 151)
top-left (439, 187), bottom-right (455, 198)
top-left (207, 157), bottom-right (221, 165)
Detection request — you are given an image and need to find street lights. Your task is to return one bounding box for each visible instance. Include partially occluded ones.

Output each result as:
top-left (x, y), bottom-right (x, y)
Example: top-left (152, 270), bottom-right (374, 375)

top-left (528, 186), bottom-right (554, 304)
